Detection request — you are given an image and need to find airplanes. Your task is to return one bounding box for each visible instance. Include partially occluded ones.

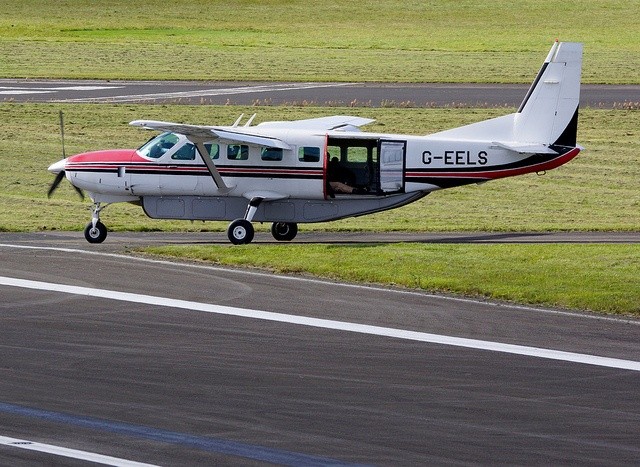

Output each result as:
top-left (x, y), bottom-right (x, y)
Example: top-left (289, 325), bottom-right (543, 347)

top-left (46, 38), bottom-right (583, 246)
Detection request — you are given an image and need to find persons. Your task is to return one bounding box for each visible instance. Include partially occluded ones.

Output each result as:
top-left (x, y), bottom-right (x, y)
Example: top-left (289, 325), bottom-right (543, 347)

top-left (327, 151), bottom-right (333, 182)
top-left (156, 138), bottom-right (183, 158)
